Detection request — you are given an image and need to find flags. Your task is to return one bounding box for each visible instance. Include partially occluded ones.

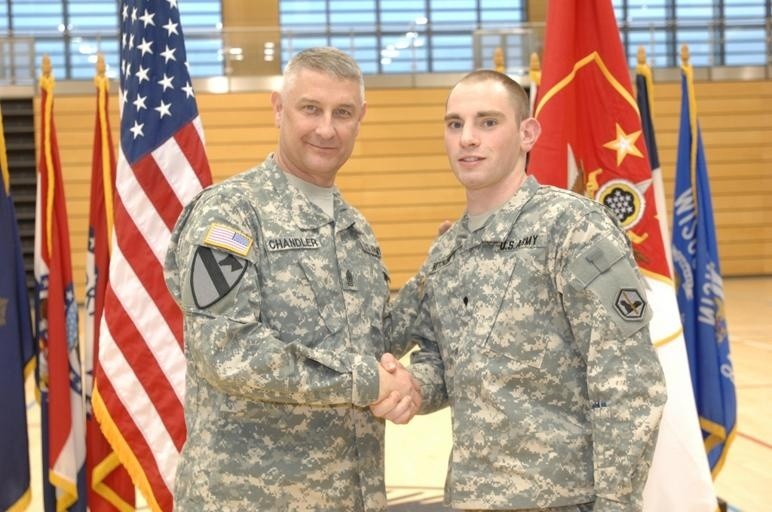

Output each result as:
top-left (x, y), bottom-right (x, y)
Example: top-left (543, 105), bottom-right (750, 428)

top-left (528, 70), bottom-right (541, 124)
top-left (86, 74), bottom-right (122, 417)
top-left (670, 64), bottom-right (738, 482)
top-left (526, 3), bottom-right (719, 510)
top-left (2, 115), bottom-right (55, 512)
top-left (33, 77), bottom-right (93, 512)
top-left (634, 65), bottom-right (675, 284)
top-left (83, 1), bottom-right (213, 512)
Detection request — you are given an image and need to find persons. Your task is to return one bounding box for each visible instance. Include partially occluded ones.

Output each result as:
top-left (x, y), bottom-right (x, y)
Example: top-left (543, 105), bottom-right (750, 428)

top-left (159, 46), bottom-right (422, 511)
top-left (366, 71), bottom-right (668, 510)
